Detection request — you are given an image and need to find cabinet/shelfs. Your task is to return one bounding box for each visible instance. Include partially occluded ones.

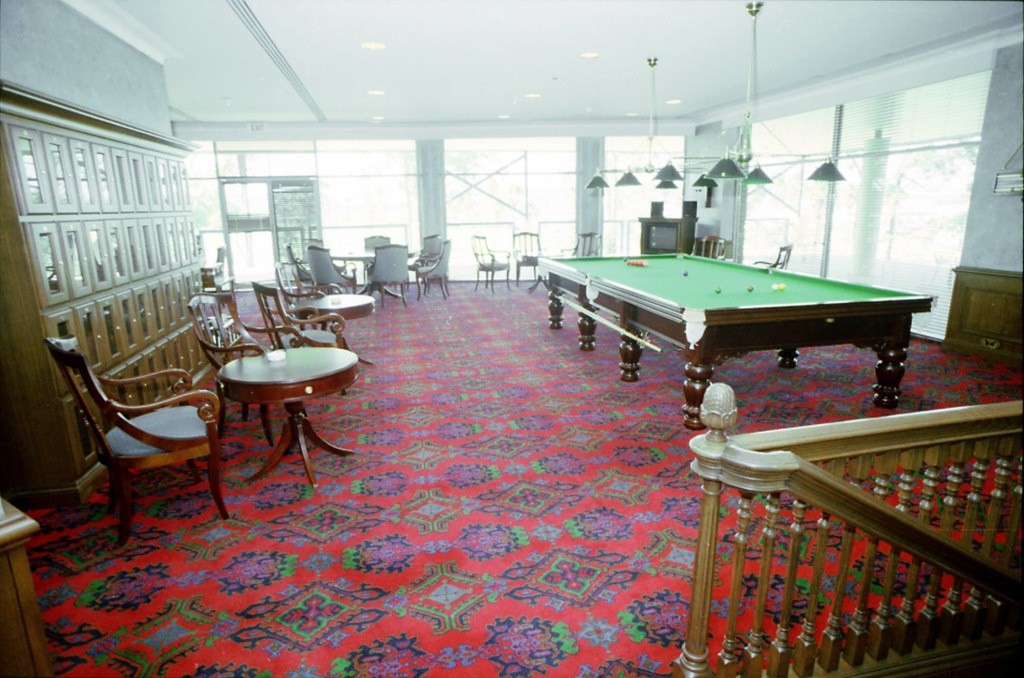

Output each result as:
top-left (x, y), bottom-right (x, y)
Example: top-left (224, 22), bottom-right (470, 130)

top-left (0, 77), bottom-right (224, 511)
top-left (941, 266), bottom-right (1024, 368)
top-left (639, 217), bottom-right (698, 257)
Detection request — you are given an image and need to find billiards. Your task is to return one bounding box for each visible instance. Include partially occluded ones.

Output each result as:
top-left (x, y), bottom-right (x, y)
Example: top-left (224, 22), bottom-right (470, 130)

top-left (624, 258), bottom-right (648, 266)
top-left (683, 272), bottom-right (688, 276)
top-left (715, 287), bottom-right (721, 294)
top-left (779, 283), bottom-right (785, 290)
top-left (748, 286), bottom-right (753, 291)
top-left (772, 284), bottom-right (778, 289)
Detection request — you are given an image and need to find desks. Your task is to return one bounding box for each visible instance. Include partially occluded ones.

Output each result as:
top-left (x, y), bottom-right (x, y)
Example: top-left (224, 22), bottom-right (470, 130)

top-left (213, 347), bottom-right (360, 490)
top-left (538, 253), bottom-right (934, 430)
top-left (330, 250), bottom-right (414, 299)
top-left (290, 293), bottom-right (376, 367)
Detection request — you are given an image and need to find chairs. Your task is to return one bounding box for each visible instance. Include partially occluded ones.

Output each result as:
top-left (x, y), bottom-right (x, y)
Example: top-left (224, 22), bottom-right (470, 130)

top-left (561, 232), bottom-right (603, 259)
top-left (43, 231), bottom-right (451, 548)
top-left (690, 235), bottom-right (725, 262)
top-left (513, 232), bottom-right (549, 287)
top-left (755, 245), bottom-right (793, 271)
top-left (469, 234), bottom-right (511, 294)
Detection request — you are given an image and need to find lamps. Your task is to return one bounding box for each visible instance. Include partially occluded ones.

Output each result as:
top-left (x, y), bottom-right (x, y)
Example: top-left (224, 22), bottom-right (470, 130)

top-left (991, 141), bottom-right (1024, 193)
top-left (584, 2), bottom-right (847, 189)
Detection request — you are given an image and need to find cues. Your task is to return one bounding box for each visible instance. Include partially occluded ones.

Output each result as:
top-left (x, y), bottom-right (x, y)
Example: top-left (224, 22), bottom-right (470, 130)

top-left (555, 294), bottom-right (664, 353)
